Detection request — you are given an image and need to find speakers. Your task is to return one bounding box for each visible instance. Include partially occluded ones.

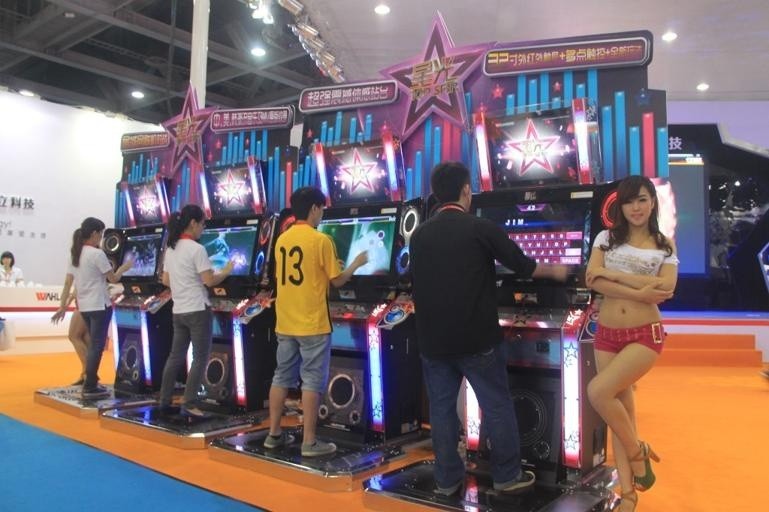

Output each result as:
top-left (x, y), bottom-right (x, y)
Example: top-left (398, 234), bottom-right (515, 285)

top-left (195, 337), bottom-right (236, 416)
top-left (315, 347), bottom-right (372, 452)
top-left (477, 368), bottom-right (566, 484)
top-left (102, 227), bottom-right (123, 279)
top-left (113, 332), bottom-right (144, 398)
top-left (394, 205), bottom-right (420, 290)
top-left (250, 211), bottom-right (280, 289)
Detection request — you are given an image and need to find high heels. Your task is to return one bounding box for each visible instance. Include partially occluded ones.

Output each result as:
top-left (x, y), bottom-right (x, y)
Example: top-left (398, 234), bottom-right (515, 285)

top-left (179, 408), bottom-right (213, 422)
top-left (617, 489), bottom-right (638, 512)
top-left (627, 440), bottom-right (660, 492)
top-left (159, 405), bottom-right (181, 417)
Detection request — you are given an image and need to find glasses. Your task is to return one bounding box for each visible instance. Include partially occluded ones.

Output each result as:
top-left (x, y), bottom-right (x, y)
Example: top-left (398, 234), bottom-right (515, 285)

top-left (200, 222), bottom-right (206, 228)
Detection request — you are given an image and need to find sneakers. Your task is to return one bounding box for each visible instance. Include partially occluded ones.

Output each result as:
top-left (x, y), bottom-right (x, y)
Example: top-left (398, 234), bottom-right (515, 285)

top-left (301, 439), bottom-right (336, 456)
top-left (71, 379), bottom-right (84, 387)
top-left (264, 431), bottom-right (295, 448)
top-left (435, 485), bottom-right (458, 495)
top-left (502, 471), bottom-right (535, 491)
top-left (82, 387), bottom-right (112, 397)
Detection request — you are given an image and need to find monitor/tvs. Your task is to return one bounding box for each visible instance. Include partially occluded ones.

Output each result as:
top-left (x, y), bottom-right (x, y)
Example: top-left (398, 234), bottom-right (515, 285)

top-left (198, 227), bottom-right (257, 277)
top-left (120, 232), bottom-right (162, 279)
top-left (477, 200), bottom-right (589, 269)
top-left (315, 214), bottom-right (396, 277)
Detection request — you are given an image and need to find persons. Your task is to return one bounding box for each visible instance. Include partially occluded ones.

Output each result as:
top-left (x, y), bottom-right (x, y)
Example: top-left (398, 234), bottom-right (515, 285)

top-left (0, 251), bottom-right (22, 283)
top-left (157, 205), bottom-right (235, 419)
top-left (53, 228), bottom-right (92, 387)
top-left (587, 176), bottom-right (679, 512)
top-left (409, 161), bottom-right (569, 495)
top-left (262, 188), bottom-right (369, 455)
top-left (56, 218), bottom-right (135, 396)
